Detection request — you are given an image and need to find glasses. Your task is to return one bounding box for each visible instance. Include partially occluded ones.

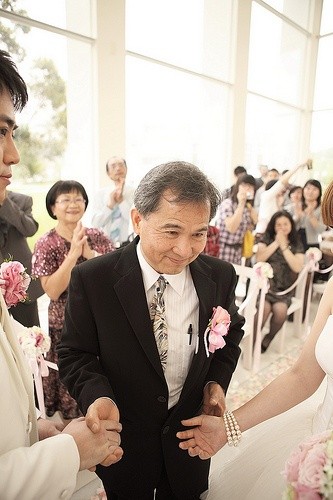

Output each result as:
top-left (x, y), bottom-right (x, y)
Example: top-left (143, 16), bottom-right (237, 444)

top-left (55, 196), bottom-right (86, 203)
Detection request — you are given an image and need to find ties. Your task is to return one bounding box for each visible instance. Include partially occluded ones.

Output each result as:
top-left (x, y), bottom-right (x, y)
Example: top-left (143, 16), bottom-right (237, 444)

top-left (149, 276), bottom-right (170, 371)
top-left (109, 205), bottom-right (121, 241)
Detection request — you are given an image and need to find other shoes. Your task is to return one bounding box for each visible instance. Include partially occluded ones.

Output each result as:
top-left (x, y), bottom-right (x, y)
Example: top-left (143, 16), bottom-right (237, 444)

top-left (261, 334), bottom-right (273, 352)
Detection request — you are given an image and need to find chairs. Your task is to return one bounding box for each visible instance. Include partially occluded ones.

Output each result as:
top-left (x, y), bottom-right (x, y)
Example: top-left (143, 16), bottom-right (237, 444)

top-left (222, 252), bottom-right (316, 370)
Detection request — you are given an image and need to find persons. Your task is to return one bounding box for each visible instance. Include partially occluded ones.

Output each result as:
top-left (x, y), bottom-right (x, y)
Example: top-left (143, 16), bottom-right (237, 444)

top-left (32, 180), bottom-right (117, 422)
top-left (92, 157), bottom-right (138, 246)
top-left (57, 161), bottom-right (245, 500)
top-left (0, 47), bottom-right (123, 500)
top-left (176, 183), bottom-right (333, 500)
top-left (201, 165), bottom-right (333, 353)
top-left (0, 191), bottom-right (46, 410)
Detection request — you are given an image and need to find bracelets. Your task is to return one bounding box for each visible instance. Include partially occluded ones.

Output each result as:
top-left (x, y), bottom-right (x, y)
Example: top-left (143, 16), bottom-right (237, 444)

top-left (223, 411), bottom-right (243, 447)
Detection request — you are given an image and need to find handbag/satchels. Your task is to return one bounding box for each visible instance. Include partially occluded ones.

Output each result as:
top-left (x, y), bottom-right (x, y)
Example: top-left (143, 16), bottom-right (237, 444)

top-left (242, 228), bottom-right (254, 257)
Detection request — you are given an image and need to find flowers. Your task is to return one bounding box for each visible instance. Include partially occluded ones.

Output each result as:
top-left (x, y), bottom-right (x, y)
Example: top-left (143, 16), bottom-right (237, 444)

top-left (204, 305), bottom-right (232, 357)
top-left (19, 325), bottom-right (53, 379)
top-left (281, 428), bottom-right (333, 500)
top-left (0, 253), bottom-right (36, 308)
